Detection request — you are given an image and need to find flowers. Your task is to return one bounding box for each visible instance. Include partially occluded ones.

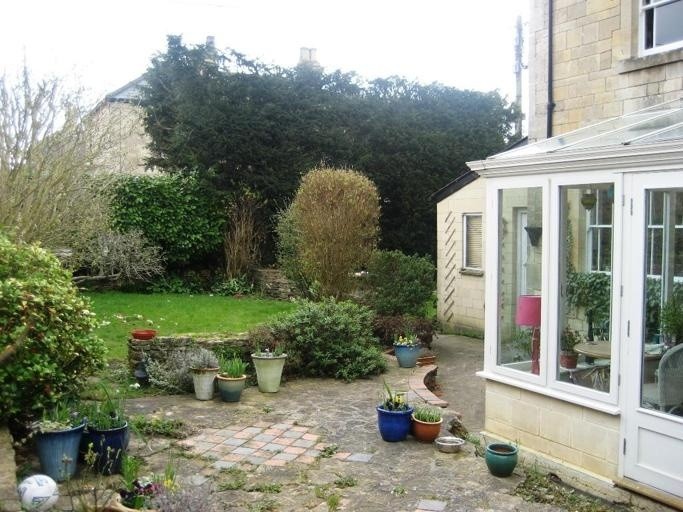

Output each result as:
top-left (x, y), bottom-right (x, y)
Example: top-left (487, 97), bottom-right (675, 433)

top-left (393, 334), bottom-right (418, 350)
top-left (117, 462), bottom-right (182, 509)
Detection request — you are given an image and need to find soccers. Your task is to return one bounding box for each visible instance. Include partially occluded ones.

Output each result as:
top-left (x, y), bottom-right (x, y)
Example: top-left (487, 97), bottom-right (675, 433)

top-left (18, 474), bottom-right (59, 512)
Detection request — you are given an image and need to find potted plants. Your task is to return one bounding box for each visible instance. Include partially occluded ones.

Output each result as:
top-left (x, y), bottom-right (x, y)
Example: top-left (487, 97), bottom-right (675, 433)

top-left (559, 325), bottom-right (578, 369)
top-left (191, 327), bottom-right (289, 402)
top-left (376, 377), bottom-right (444, 443)
top-left (30, 383), bottom-right (132, 482)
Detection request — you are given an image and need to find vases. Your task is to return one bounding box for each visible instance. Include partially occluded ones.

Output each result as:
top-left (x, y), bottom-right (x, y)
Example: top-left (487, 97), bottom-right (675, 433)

top-left (393, 344), bottom-right (419, 368)
top-left (485, 443), bottom-right (518, 478)
top-left (435, 436), bottom-right (466, 453)
top-left (130, 330), bottom-right (158, 339)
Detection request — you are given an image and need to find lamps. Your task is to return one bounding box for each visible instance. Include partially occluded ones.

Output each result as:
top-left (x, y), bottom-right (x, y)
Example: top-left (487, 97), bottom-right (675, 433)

top-left (514, 295), bottom-right (541, 375)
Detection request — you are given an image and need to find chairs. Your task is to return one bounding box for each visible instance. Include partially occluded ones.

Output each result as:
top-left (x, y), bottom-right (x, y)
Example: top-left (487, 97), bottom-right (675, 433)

top-left (641, 342), bottom-right (683, 414)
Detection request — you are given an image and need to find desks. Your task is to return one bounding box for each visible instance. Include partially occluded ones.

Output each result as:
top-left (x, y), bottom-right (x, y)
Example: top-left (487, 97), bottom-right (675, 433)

top-left (572, 340), bottom-right (669, 391)
